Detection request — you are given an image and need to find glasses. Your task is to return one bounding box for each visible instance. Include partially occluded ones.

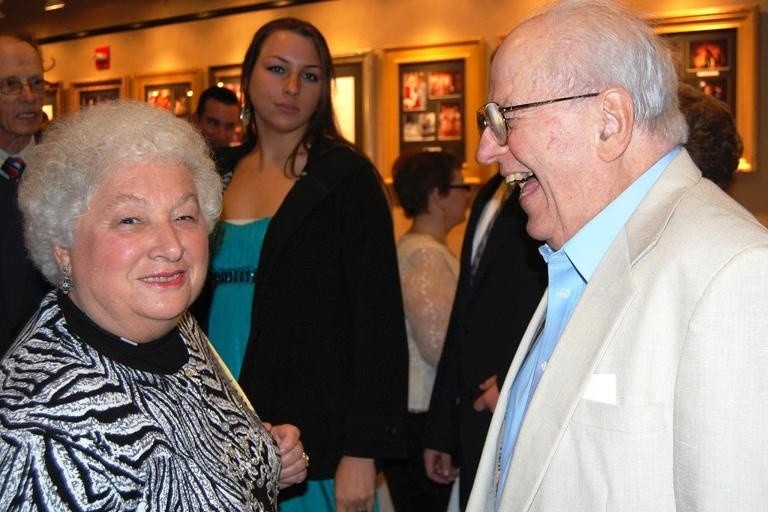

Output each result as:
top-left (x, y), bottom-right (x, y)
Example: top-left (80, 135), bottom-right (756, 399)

top-left (0, 78), bottom-right (51, 96)
top-left (449, 184), bottom-right (471, 191)
top-left (477, 93), bottom-right (600, 146)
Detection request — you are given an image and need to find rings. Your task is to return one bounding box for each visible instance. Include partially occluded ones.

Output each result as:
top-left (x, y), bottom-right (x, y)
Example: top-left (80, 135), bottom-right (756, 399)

top-left (302, 452), bottom-right (310, 467)
top-left (357, 506), bottom-right (365, 512)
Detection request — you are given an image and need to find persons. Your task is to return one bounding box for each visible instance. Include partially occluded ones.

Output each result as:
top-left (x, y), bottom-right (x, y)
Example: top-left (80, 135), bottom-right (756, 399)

top-left (191, 87), bottom-right (241, 147)
top-left (677, 80), bottom-right (744, 191)
top-left (422, 171), bottom-right (548, 512)
top-left (466, 0), bottom-right (768, 512)
top-left (190, 18), bottom-right (409, 512)
top-left (0, 99), bottom-right (308, 512)
top-left (0, 32), bottom-right (57, 359)
top-left (382, 151), bottom-right (471, 512)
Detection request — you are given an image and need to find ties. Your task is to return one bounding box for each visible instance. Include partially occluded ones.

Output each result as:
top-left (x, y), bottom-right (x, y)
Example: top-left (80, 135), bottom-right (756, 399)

top-left (470, 182), bottom-right (516, 287)
top-left (0, 156), bottom-right (25, 184)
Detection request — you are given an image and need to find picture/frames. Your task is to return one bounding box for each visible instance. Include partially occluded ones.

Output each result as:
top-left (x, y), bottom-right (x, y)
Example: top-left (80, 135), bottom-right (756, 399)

top-left (44, 82), bottom-right (62, 124)
top-left (327, 49), bottom-right (376, 170)
top-left (379, 36), bottom-right (490, 179)
top-left (205, 62), bottom-right (248, 116)
top-left (65, 73), bottom-right (129, 115)
top-left (134, 68), bottom-right (203, 126)
top-left (639, 3), bottom-right (758, 174)
